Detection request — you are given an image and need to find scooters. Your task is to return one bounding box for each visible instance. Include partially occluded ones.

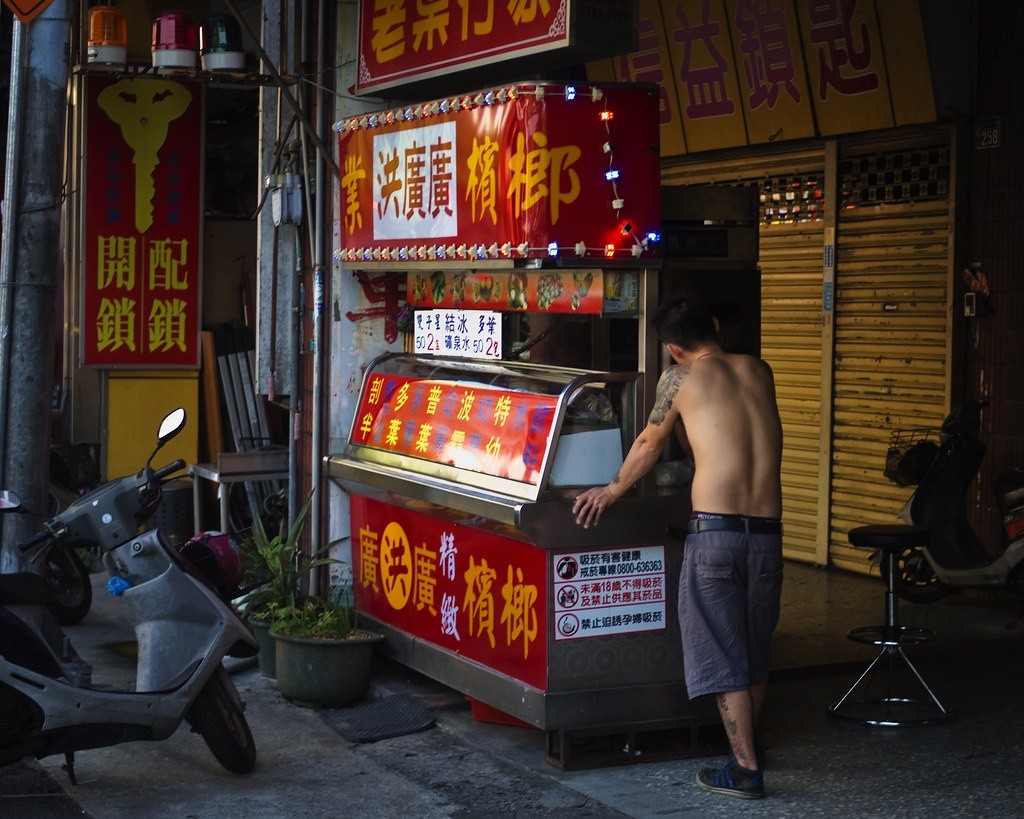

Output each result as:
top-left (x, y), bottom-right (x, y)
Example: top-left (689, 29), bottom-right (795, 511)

top-left (2, 407), bottom-right (257, 785)
top-left (878, 399), bottom-right (1024, 606)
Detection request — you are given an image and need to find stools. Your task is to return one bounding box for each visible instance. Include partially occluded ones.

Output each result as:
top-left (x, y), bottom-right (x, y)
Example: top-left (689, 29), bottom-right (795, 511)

top-left (826, 520), bottom-right (954, 732)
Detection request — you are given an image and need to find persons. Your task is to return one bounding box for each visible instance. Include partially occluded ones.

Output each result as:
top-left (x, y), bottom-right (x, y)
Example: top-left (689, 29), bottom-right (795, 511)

top-left (571, 294), bottom-right (784, 796)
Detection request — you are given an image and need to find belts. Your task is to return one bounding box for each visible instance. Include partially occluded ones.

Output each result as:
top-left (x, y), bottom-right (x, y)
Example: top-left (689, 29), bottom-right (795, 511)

top-left (686, 518), bottom-right (782, 535)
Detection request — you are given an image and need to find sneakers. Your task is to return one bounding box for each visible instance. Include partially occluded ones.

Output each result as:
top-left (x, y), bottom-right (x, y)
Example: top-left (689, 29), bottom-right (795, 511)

top-left (694, 760), bottom-right (764, 800)
top-left (755, 749), bottom-right (771, 767)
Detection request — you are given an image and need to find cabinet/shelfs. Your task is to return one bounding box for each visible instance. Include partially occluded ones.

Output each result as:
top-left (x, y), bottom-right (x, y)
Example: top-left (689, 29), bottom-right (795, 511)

top-left (328, 347), bottom-right (681, 772)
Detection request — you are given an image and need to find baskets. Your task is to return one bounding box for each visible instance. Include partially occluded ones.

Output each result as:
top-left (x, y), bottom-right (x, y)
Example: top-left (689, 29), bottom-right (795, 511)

top-left (882, 428), bottom-right (946, 488)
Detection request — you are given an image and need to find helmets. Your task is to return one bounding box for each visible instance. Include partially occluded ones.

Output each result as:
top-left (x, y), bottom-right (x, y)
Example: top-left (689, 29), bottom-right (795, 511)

top-left (178, 529), bottom-right (244, 595)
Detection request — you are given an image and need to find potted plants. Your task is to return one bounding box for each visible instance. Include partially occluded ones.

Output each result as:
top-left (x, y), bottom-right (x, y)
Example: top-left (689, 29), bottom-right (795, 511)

top-left (221, 478), bottom-right (354, 677)
top-left (263, 581), bottom-right (386, 710)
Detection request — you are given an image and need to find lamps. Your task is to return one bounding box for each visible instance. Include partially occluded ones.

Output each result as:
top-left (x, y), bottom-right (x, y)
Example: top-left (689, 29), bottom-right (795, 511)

top-left (151, 13), bottom-right (199, 72)
top-left (197, 9), bottom-right (247, 73)
top-left (84, 6), bottom-right (131, 68)
